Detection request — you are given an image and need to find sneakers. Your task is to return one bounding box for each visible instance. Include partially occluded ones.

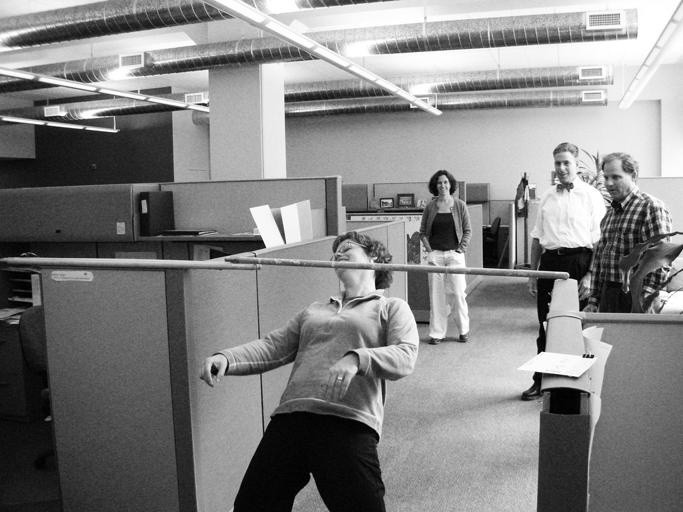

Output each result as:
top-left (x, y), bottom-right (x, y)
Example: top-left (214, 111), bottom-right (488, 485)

top-left (459, 332), bottom-right (470, 342)
top-left (522, 382), bottom-right (542, 400)
top-left (430, 337), bottom-right (446, 344)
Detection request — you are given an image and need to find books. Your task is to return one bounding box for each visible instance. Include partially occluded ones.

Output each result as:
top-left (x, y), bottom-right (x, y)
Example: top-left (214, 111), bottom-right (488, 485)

top-left (158, 228), bottom-right (216, 237)
top-left (139, 191), bottom-right (175, 235)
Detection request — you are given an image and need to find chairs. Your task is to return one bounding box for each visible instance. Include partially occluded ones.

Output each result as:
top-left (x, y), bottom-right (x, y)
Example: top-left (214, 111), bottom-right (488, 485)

top-left (16, 303), bottom-right (56, 473)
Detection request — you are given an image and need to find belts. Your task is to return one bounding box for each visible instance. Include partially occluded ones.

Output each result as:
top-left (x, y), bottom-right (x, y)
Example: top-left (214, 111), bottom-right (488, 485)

top-left (546, 247), bottom-right (579, 257)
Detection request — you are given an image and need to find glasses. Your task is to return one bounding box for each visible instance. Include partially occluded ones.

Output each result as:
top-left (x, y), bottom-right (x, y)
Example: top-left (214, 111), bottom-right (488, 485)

top-left (329, 241), bottom-right (365, 260)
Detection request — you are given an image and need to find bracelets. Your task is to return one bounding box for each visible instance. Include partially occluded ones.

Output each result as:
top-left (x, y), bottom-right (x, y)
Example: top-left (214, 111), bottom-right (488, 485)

top-left (588, 301), bottom-right (599, 306)
top-left (587, 269), bottom-right (592, 273)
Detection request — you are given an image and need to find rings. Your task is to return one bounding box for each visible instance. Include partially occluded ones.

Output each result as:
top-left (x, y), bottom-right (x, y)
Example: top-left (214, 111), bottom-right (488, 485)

top-left (335, 376), bottom-right (343, 383)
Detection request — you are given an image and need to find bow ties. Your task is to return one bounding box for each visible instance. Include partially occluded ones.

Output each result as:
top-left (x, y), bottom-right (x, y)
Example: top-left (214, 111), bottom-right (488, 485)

top-left (557, 182), bottom-right (574, 190)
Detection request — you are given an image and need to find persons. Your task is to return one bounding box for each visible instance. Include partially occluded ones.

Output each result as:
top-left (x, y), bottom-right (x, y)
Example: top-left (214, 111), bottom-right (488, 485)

top-left (521, 143), bottom-right (607, 399)
top-left (582, 152), bottom-right (672, 312)
top-left (199, 230), bottom-right (418, 511)
top-left (418, 170), bottom-right (471, 344)
